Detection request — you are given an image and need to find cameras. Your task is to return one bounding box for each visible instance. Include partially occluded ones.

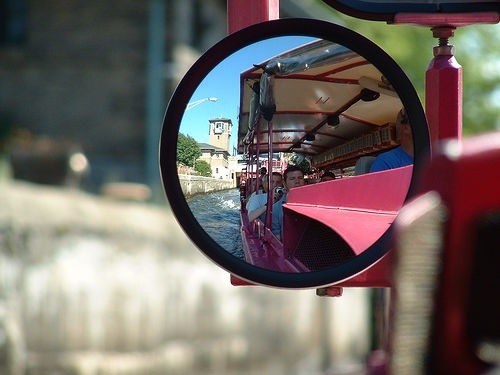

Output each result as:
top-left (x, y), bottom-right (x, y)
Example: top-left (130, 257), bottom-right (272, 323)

top-left (276, 187), bottom-right (288, 195)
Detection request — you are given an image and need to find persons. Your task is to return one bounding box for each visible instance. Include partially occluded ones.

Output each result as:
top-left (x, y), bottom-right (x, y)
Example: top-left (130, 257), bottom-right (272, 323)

top-left (246, 174), bottom-right (268, 211)
top-left (248, 172), bottom-right (282, 222)
top-left (272, 166), bottom-right (304, 240)
top-left (369, 107), bottom-right (414, 172)
top-left (321, 172), bottom-right (335, 182)
